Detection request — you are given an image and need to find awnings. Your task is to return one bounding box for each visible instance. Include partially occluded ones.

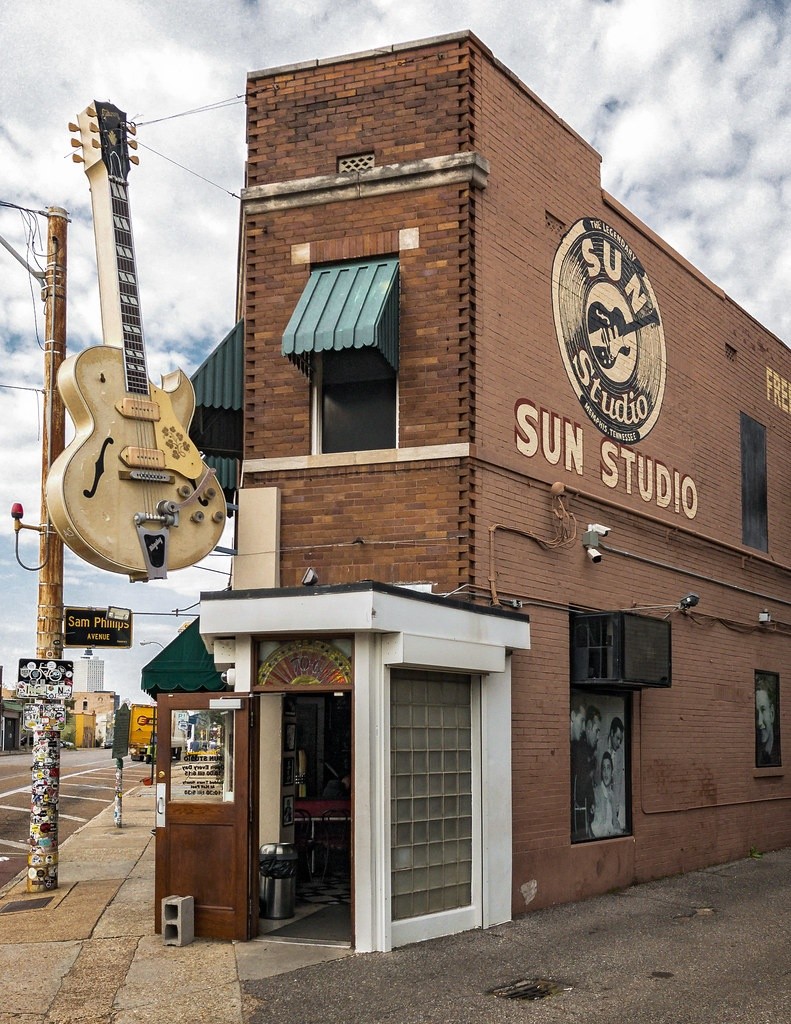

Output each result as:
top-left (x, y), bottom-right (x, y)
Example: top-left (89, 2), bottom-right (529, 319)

top-left (189, 319), bottom-right (244, 518)
top-left (282, 259), bottom-right (398, 378)
top-left (141, 617), bottom-right (228, 701)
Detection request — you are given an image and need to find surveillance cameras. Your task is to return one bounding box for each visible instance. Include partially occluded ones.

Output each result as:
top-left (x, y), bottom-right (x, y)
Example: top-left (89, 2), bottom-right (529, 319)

top-left (587, 548), bottom-right (602, 564)
top-left (592, 523), bottom-right (611, 538)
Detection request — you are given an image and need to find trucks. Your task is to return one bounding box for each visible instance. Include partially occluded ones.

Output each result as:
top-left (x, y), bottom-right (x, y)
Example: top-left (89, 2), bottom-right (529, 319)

top-left (131, 703), bottom-right (180, 761)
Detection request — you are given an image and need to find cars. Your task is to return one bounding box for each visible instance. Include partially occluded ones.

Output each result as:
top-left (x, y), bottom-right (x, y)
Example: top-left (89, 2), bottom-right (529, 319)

top-left (104, 739), bottom-right (114, 748)
top-left (20, 735), bottom-right (33, 745)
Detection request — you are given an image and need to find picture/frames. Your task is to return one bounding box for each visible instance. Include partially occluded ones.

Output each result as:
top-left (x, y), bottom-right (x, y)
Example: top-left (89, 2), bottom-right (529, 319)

top-left (283, 794), bottom-right (295, 826)
top-left (283, 757), bottom-right (294, 786)
top-left (285, 722), bottom-right (295, 751)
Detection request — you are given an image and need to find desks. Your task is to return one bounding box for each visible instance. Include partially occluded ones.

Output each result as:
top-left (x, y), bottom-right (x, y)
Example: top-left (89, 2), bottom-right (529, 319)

top-left (294, 799), bottom-right (351, 864)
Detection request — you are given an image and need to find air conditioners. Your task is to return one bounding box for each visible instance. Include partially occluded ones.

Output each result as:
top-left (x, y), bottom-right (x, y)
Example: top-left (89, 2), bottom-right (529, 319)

top-left (571, 610), bottom-right (672, 690)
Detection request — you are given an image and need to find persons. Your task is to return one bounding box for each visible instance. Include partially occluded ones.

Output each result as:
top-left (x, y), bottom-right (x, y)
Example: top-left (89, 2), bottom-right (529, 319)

top-left (755, 677), bottom-right (781, 767)
top-left (570, 698), bottom-right (627, 838)
top-left (283, 799), bottom-right (292, 823)
top-left (321, 748), bottom-right (350, 787)
top-left (190, 738), bottom-right (218, 753)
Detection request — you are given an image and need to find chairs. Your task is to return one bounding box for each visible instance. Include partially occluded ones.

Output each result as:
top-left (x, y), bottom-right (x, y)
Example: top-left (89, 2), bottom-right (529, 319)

top-left (320, 807), bottom-right (351, 883)
top-left (294, 808), bottom-right (314, 882)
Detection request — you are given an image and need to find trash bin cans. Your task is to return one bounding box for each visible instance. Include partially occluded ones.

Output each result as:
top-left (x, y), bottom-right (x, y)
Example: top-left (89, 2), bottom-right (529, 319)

top-left (258, 842), bottom-right (299, 920)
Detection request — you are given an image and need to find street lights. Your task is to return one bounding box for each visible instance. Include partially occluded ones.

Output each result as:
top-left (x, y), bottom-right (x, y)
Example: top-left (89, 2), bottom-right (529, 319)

top-left (140, 640), bottom-right (165, 650)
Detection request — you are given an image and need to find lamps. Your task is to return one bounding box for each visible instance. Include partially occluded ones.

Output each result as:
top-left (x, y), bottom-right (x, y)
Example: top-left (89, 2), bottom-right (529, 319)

top-left (302, 568), bottom-right (319, 585)
top-left (679, 592), bottom-right (700, 608)
top-left (106, 606), bottom-right (132, 621)
